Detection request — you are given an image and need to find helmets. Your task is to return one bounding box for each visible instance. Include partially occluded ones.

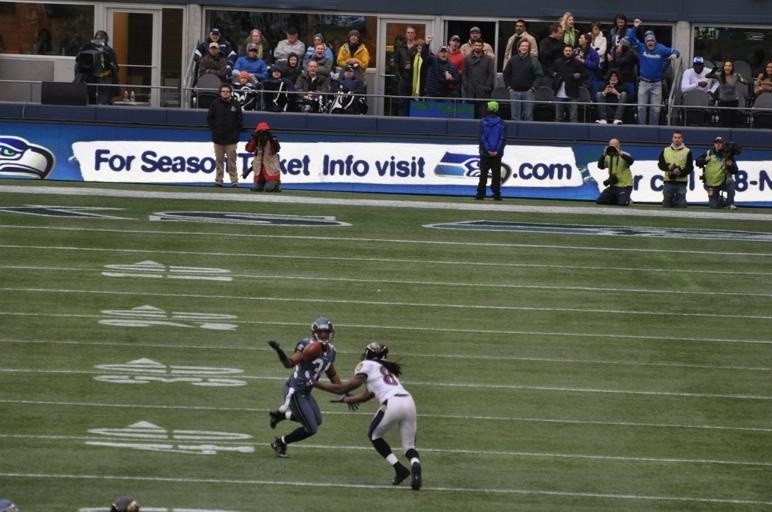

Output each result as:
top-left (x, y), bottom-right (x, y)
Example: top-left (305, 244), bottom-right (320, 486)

top-left (365, 342), bottom-right (388, 360)
top-left (311, 318), bottom-right (335, 345)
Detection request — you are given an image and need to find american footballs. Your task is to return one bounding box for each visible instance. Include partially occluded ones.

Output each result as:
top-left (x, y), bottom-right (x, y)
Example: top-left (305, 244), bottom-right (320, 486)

top-left (303, 342), bottom-right (322, 362)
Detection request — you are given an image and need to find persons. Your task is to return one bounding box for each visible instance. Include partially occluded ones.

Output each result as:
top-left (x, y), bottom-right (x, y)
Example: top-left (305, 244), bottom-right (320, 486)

top-left (205, 84), bottom-right (244, 188)
top-left (696, 136), bottom-right (740, 211)
top-left (502, 11), bottom-right (680, 126)
top-left (298, 339), bottom-right (422, 490)
top-left (245, 120), bottom-right (282, 193)
top-left (681, 56), bottom-right (771, 127)
top-left (474, 100), bottom-right (508, 202)
top-left (657, 129), bottom-right (694, 208)
top-left (192, 28), bottom-right (375, 114)
top-left (385, 24), bottom-right (496, 120)
top-left (32, 24), bottom-right (91, 55)
top-left (0, 498), bottom-right (20, 511)
top-left (267, 316), bottom-right (360, 460)
top-left (596, 137), bottom-right (636, 207)
top-left (109, 495), bottom-right (140, 510)
top-left (80, 30), bottom-right (122, 105)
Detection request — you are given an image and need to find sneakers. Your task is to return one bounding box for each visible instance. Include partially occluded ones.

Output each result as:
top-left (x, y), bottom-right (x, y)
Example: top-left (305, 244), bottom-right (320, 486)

top-left (392, 462), bottom-right (422, 491)
top-left (269, 410), bottom-right (281, 428)
top-left (271, 439), bottom-right (288, 458)
top-left (595, 119), bottom-right (607, 124)
top-left (613, 119), bottom-right (622, 124)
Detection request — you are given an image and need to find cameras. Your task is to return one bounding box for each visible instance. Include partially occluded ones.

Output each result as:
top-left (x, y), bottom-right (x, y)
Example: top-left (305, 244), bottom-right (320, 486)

top-left (604, 175), bottom-right (618, 186)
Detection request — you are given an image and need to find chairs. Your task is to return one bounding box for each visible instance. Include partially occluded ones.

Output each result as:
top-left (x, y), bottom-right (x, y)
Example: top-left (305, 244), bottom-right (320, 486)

top-left (193, 38), bottom-right (771, 129)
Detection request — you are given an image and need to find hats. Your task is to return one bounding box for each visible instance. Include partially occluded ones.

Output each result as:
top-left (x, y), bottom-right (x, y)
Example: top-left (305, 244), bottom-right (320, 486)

top-left (644, 35), bottom-right (656, 41)
top-left (693, 57), bottom-right (704, 64)
top-left (621, 37), bottom-right (631, 46)
top-left (487, 101), bottom-right (499, 112)
